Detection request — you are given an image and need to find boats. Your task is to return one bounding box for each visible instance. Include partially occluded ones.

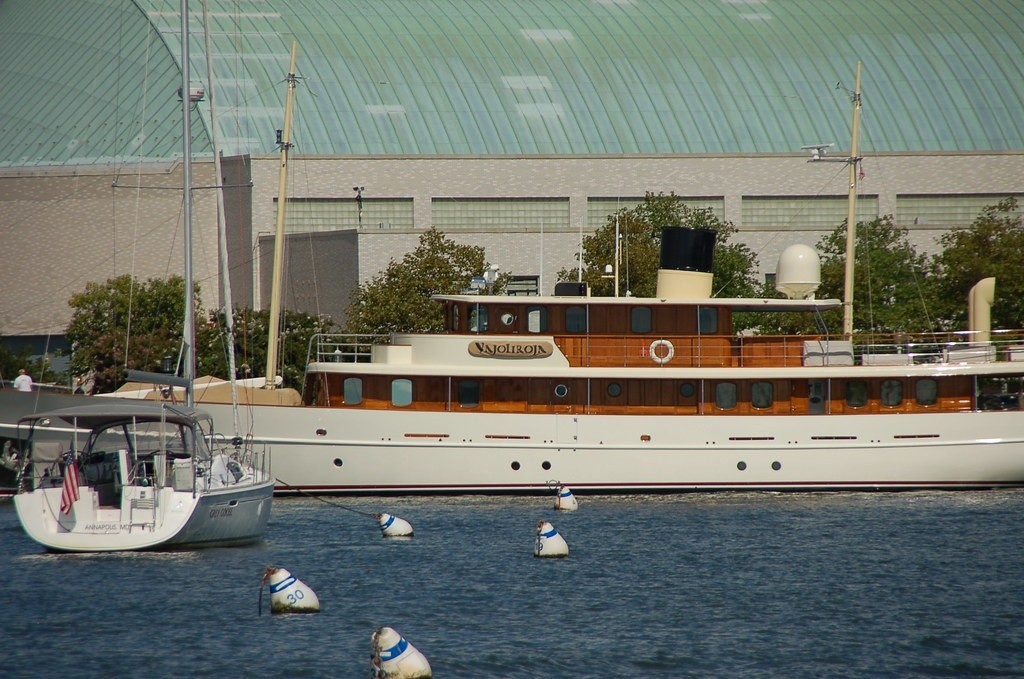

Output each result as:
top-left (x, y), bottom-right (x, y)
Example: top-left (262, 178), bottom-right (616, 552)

top-left (1, 58), bottom-right (1024, 497)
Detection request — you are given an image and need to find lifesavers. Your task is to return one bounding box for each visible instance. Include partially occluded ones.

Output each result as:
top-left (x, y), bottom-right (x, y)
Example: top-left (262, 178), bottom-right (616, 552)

top-left (648, 340), bottom-right (676, 363)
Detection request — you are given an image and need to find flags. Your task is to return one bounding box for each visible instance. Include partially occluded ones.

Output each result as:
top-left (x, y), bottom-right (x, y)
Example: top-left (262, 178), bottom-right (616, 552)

top-left (59, 440), bottom-right (81, 515)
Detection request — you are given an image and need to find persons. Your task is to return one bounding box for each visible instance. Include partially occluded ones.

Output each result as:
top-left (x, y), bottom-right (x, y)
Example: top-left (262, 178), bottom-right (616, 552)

top-left (14, 369), bottom-right (32, 392)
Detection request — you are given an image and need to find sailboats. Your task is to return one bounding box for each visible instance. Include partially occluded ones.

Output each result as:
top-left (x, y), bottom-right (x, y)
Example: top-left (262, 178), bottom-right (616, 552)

top-left (1, 3), bottom-right (277, 553)
